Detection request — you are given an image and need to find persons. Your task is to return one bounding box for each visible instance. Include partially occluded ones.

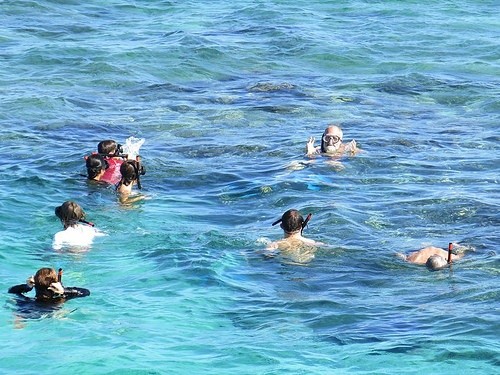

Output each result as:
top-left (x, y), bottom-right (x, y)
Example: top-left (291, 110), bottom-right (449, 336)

top-left (283, 125), bottom-right (364, 170)
top-left (84, 139), bottom-right (125, 191)
top-left (63, 154), bottom-right (118, 202)
top-left (394, 241), bottom-right (466, 271)
top-left (255, 209), bottom-right (323, 281)
top-left (6, 268), bottom-right (90, 331)
top-left (117, 159), bottom-right (151, 209)
top-left (52, 202), bottom-right (108, 263)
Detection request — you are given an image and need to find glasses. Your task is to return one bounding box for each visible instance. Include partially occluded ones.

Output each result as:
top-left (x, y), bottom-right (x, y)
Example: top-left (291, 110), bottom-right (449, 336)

top-left (323, 135), bottom-right (340, 144)
